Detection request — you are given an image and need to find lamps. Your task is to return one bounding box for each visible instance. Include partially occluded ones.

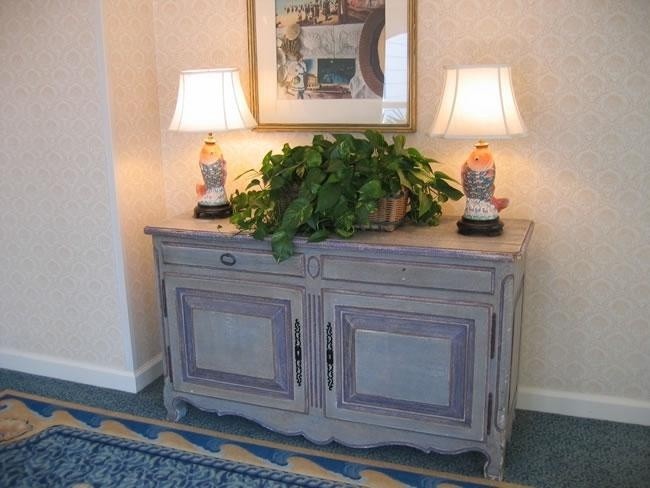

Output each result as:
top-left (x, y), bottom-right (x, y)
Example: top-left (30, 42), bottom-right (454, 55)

top-left (168, 67), bottom-right (258, 219)
top-left (425, 63), bottom-right (530, 237)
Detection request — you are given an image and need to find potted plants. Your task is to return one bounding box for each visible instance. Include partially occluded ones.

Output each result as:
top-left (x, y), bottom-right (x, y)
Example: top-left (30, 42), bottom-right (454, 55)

top-left (229, 129), bottom-right (465, 264)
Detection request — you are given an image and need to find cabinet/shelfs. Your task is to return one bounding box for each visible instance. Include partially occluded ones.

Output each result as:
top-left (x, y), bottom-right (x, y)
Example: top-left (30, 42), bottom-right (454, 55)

top-left (143, 204), bottom-right (535, 482)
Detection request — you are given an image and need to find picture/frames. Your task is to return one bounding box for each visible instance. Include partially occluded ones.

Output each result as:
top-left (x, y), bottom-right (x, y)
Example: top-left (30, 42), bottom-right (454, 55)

top-left (246, 0), bottom-right (418, 133)
